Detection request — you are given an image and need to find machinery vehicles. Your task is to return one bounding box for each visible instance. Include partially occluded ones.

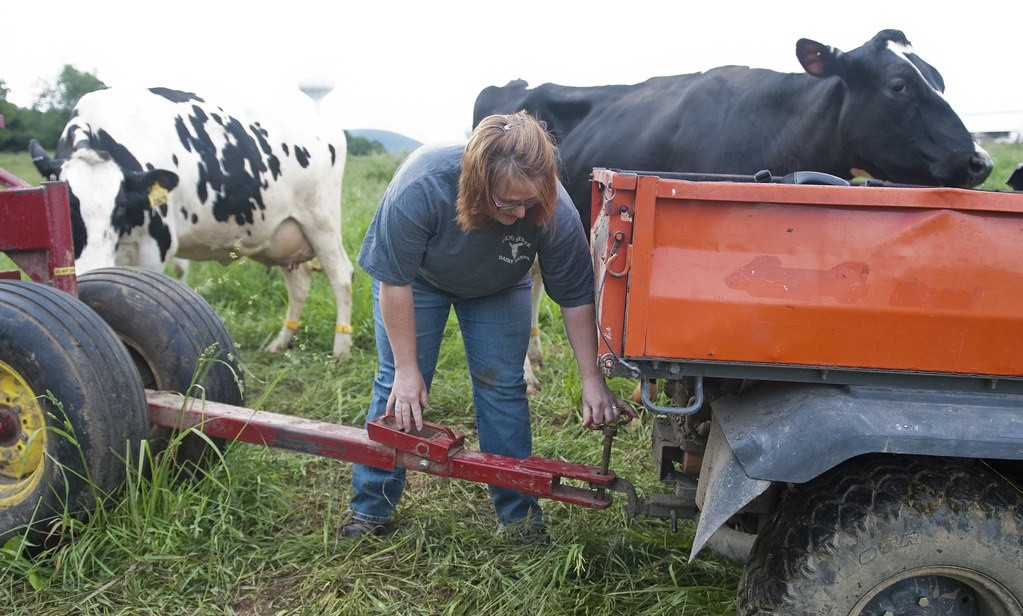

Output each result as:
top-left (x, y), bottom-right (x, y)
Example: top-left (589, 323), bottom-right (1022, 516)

top-left (587, 167), bottom-right (1023, 616)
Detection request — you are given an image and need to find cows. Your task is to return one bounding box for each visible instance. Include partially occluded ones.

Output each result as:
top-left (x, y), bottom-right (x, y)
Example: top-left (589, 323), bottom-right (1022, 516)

top-left (28, 87), bottom-right (354, 364)
top-left (472, 25), bottom-right (993, 401)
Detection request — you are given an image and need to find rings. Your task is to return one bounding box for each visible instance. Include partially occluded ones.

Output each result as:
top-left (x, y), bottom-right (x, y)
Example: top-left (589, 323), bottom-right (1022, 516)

top-left (612, 406), bottom-right (619, 409)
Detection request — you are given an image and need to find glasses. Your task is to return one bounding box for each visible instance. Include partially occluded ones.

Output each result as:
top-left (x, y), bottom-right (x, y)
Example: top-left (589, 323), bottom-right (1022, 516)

top-left (487, 191), bottom-right (540, 211)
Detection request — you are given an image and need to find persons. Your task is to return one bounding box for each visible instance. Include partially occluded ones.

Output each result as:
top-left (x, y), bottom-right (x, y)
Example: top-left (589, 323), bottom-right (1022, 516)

top-left (338, 110), bottom-right (633, 538)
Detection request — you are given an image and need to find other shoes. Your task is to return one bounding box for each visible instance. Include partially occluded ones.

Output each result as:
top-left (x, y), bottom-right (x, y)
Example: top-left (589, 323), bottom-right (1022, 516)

top-left (337, 517), bottom-right (392, 539)
top-left (497, 520), bottom-right (550, 545)
